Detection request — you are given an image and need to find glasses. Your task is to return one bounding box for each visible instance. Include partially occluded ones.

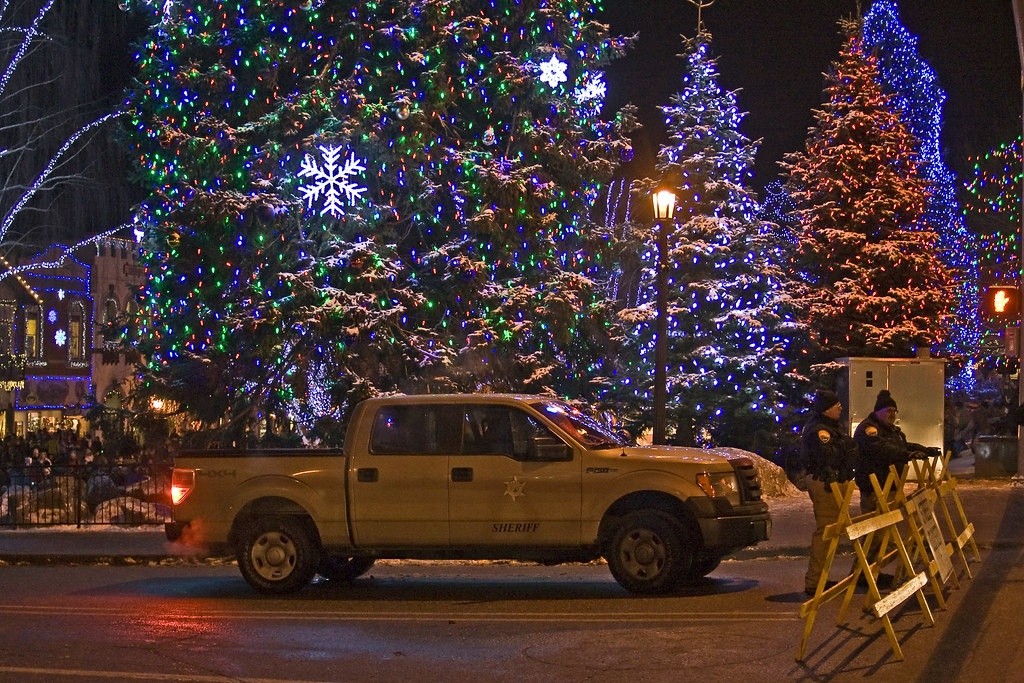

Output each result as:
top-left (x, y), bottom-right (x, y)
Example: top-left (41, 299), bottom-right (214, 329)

top-left (832, 403), bottom-right (841, 408)
top-left (878, 407), bottom-right (898, 415)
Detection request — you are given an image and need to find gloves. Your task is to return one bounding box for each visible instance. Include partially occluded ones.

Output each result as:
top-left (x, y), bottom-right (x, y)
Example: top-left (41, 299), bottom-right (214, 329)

top-left (925, 447), bottom-right (941, 457)
top-left (906, 450), bottom-right (928, 460)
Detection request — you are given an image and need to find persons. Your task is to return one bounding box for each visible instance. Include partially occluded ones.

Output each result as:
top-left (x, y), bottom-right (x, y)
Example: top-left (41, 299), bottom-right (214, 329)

top-left (849, 389), bottom-right (942, 588)
top-left (946, 389), bottom-right (1024, 457)
top-left (0, 422), bottom-right (302, 489)
top-left (801, 391), bottom-right (858, 594)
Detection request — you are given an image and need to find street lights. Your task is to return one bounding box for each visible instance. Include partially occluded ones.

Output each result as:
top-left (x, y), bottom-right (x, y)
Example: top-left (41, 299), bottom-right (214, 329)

top-left (649, 173), bottom-right (677, 446)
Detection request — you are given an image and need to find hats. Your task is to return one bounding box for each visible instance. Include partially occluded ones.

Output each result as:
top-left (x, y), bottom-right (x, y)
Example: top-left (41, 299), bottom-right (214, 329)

top-left (811, 390), bottom-right (839, 413)
top-left (874, 390), bottom-right (897, 412)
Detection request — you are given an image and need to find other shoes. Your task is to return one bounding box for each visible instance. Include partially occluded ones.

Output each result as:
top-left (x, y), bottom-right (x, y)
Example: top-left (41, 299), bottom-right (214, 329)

top-left (849, 572), bottom-right (894, 589)
top-left (805, 580), bottom-right (839, 596)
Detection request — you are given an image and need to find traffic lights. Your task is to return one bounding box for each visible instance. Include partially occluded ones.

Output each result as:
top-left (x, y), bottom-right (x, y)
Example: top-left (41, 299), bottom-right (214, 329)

top-left (986, 285), bottom-right (1020, 319)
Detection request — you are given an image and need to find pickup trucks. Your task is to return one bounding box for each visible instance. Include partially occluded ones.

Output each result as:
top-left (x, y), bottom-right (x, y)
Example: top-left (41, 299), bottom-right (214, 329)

top-left (164, 398), bottom-right (773, 597)
